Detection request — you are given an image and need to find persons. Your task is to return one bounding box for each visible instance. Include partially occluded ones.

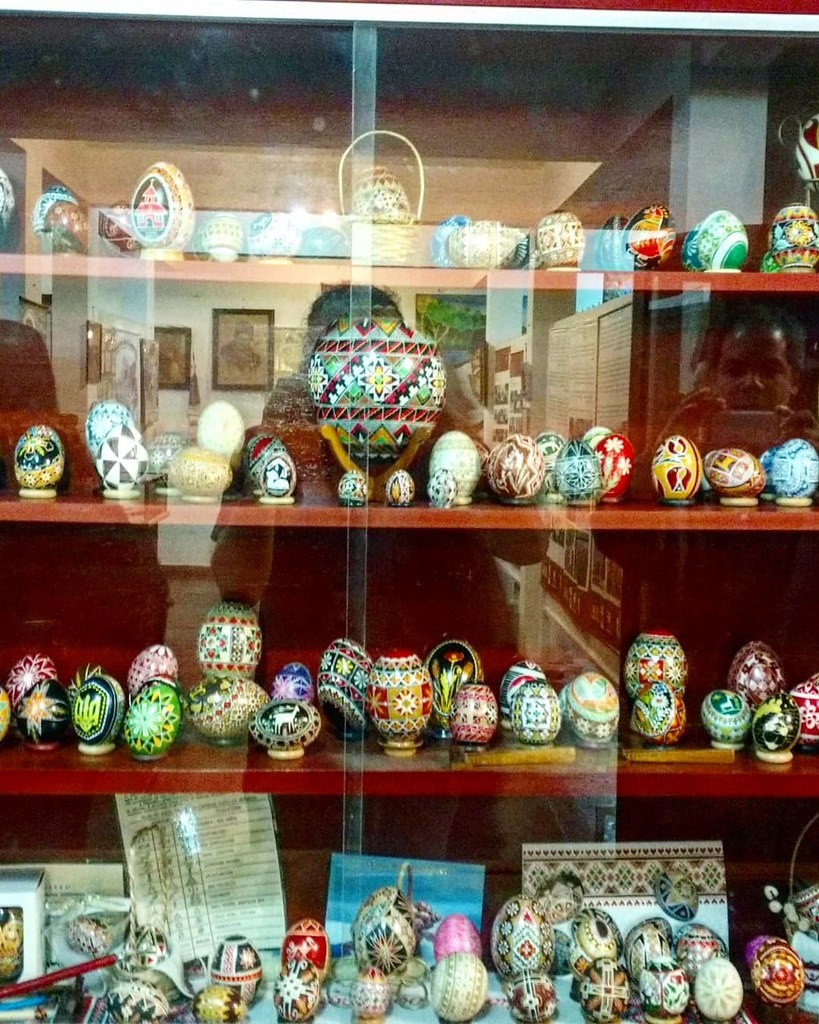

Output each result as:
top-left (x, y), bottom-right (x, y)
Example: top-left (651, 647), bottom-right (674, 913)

top-left (581, 300), bottom-right (819, 977)
top-left (0, 318), bottom-right (174, 856)
top-left (210, 284), bottom-right (551, 983)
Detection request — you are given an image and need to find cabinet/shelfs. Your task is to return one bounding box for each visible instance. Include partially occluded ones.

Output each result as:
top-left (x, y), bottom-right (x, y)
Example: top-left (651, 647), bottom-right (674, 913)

top-left (0, 0), bottom-right (819, 1024)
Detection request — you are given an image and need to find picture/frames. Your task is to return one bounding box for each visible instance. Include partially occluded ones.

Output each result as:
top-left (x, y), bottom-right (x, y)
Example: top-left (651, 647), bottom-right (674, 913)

top-left (86, 320), bottom-right (103, 385)
top-left (140, 338), bottom-right (158, 434)
top-left (212, 308), bottom-right (275, 393)
top-left (153, 326), bottom-right (194, 392)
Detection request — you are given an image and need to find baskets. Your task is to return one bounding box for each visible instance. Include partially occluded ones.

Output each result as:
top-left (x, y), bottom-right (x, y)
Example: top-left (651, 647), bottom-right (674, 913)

top-left (337, 130), bottom-right (425, 268)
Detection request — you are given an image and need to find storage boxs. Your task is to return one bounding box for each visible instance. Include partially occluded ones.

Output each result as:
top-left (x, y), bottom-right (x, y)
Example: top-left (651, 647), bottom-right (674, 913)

top-left (1, 868), bottom-right (47, 988)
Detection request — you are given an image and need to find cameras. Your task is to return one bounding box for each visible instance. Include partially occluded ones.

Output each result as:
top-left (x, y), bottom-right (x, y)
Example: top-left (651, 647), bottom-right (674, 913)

top-left (716, 410), bottom-right (779, 433)
